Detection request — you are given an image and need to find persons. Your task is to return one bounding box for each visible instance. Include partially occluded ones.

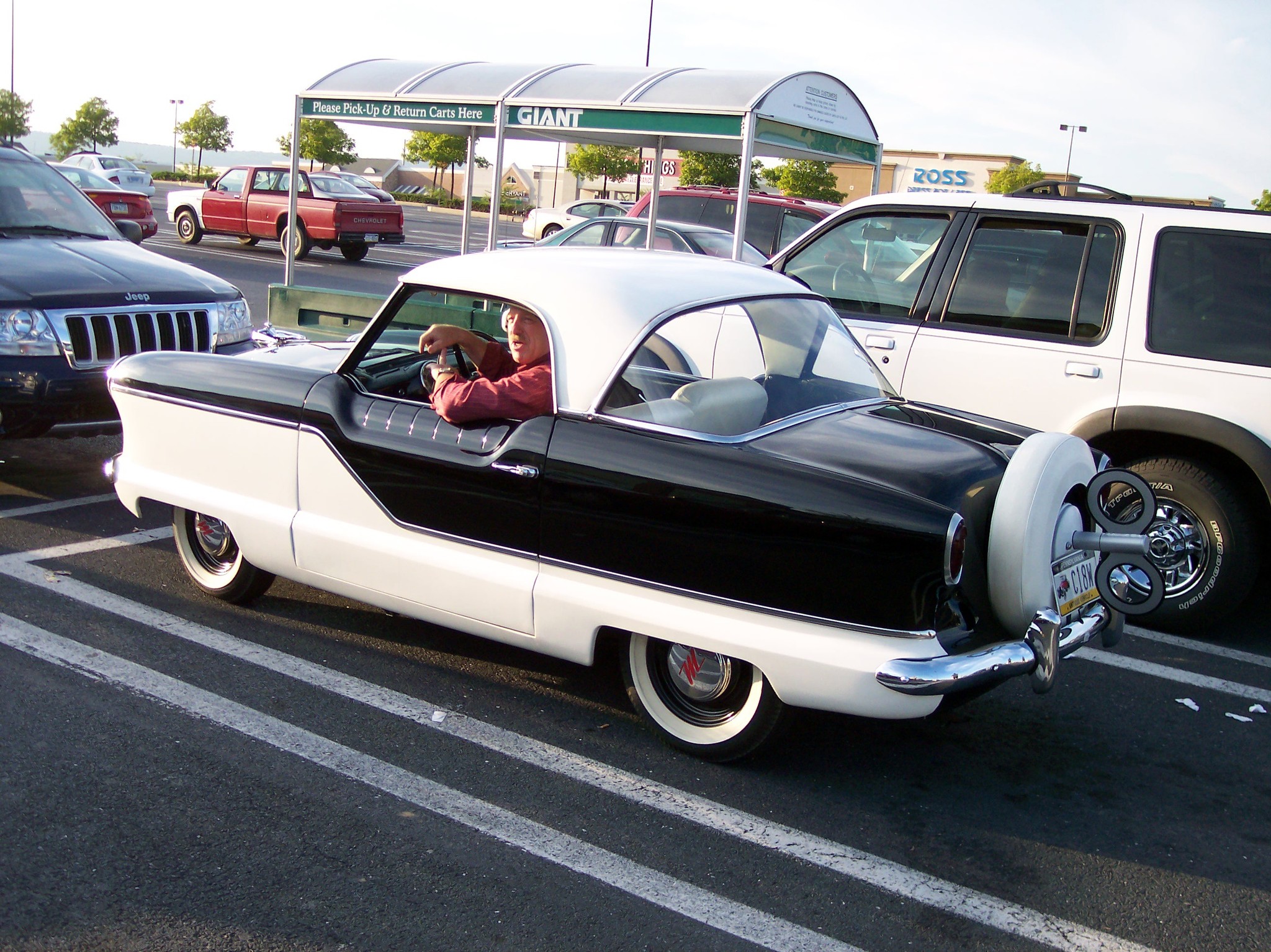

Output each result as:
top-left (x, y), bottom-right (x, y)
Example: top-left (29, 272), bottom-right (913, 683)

top-left (998, 249), bottom-right (1083, 338)
top-left (419, 306), bottom-right (553, 427)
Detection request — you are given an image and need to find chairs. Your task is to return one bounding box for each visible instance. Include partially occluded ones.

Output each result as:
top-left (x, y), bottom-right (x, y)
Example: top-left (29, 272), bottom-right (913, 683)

top-left (948, 258), bottom-right (1074, 318)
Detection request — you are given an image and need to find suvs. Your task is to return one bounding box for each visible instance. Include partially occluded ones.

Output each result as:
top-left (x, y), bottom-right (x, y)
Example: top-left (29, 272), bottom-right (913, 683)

top-left (0, 141), bottom-right (262, 447)
top-left (741, 180), bottom-right (1271, 641)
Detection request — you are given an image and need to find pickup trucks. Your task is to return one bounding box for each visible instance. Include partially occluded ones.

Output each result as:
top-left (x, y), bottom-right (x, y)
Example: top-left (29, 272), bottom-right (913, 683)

top-left (167, 164), bottom-right (406, 262)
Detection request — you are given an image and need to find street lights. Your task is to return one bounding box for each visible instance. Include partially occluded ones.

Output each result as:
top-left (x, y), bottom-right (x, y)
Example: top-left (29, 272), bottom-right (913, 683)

top-left (169, 99), bottom-right (183, 173)
top-left (1059, 124), bottom-right (1088, 196)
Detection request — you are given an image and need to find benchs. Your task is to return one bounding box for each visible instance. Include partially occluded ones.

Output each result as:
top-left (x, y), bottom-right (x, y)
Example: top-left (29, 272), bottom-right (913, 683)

top-left (612, 378), bottom-right (769, 436)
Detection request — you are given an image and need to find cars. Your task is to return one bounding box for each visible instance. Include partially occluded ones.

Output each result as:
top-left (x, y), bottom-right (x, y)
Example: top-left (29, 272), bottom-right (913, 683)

top-left (98, 241), bottom-right (1166, 772)
top-left (481, 184), bottom-right (932, 307)
top-left (60, 152), bottom-right (156, 201)
top-left (253, 170), bottom-right (395, 203)
top-left (19, 159), bottom-right (158, 246)
